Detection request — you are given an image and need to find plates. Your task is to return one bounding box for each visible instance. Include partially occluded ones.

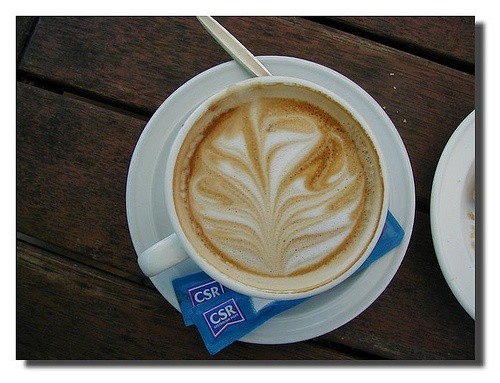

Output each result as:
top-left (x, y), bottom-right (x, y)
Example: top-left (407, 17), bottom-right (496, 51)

top-left (126, 54), bottom-right (414, 344)
top-left (429, 108), bottom-right (474, 324)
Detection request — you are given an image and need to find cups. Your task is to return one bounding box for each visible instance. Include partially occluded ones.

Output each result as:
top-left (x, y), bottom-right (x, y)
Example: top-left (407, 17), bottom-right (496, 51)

top-left (136, 76), bottom-right (389, 301)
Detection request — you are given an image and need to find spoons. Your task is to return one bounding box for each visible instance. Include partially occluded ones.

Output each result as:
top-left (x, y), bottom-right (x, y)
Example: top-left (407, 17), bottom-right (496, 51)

top-left (196, 16), bottom-right (274, 78)
